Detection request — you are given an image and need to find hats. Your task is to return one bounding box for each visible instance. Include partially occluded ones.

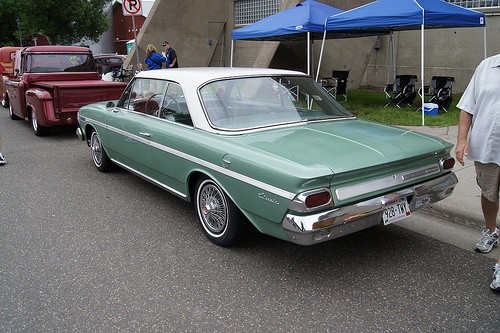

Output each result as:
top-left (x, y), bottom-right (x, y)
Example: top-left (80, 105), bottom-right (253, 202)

top-left (162, 40), bottom-right (169, 46)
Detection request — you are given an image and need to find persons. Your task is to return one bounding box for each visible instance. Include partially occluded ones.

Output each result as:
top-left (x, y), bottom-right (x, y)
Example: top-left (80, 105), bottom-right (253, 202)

top-left (455, 54), bottom-right (500, 291)
top-left (162, 41), bottom-right (179, 68)
top-left (144, 44), bottom-right (166, 70)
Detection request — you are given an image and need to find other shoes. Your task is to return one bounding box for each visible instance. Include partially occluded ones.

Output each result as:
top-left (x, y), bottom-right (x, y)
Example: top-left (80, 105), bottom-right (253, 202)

top-left (0, 153), bottom-right (7, 163)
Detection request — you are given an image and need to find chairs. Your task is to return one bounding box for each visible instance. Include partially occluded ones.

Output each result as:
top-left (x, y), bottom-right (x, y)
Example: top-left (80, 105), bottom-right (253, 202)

top-left (416, 76), bottom-right (455, 113)
top-left (383, 75), bottom-right (418, 110)
top-left (320, 70), bottom-right (349, 102)
top-left (279, 78), bottom-right (307, 102)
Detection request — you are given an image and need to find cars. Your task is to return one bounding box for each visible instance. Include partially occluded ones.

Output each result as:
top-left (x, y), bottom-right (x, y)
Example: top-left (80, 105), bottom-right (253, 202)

top-left (0, 46), bottom-right (21, 109)
top-left (76, 67), bottom-right (459, 248)
top-left (93, 54), bottom-right (126, 73)
top-left (5, 46), bottom-right (158, 136)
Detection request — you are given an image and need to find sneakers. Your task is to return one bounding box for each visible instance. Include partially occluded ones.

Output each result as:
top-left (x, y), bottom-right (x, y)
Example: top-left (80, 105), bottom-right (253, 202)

top-left (474, 226), bottom-right (500, 253)
top-left (490, 262), bottom-right (500, 290)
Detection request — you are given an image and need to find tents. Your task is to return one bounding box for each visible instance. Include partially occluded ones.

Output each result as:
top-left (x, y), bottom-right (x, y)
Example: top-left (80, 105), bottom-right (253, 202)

top-left (230, 0), bottom-right (486, 126)
